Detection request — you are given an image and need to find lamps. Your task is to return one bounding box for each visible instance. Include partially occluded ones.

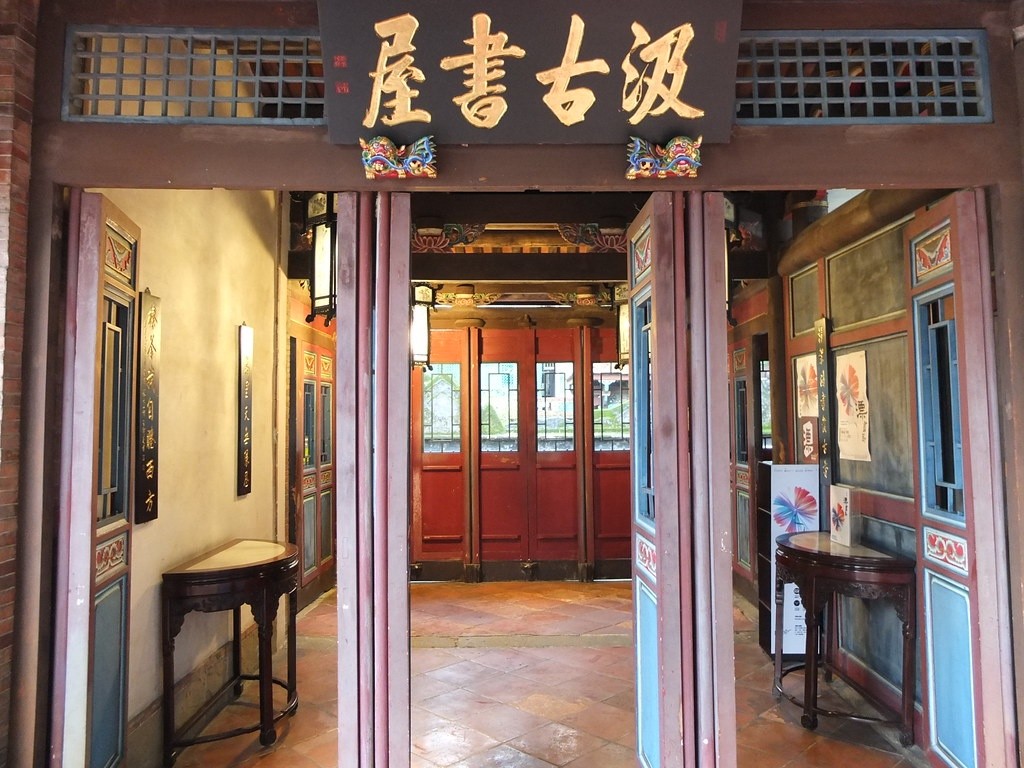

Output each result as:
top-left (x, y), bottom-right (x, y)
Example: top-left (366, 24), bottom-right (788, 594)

top-left (602, 282), bottom-right (629, 370)
top-left (724, 197), bottom-right (739, 326)
top-left (289, 190), bottom-right (337, 327)
top-left (407, 280), bottom-right (444, 373)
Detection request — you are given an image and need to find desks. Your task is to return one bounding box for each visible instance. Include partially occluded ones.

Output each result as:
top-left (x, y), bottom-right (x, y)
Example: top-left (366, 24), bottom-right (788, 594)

top-left (161, 536), bottom-right (301, 766)
top-left (773, 530), bottom-right (916, 747)
